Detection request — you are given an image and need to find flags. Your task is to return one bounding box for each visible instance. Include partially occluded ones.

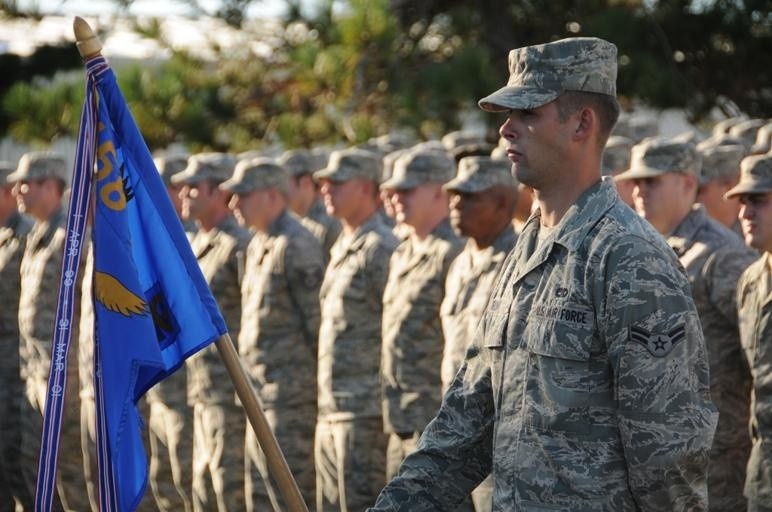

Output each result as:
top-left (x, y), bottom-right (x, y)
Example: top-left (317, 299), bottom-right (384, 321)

top-left (92, 74), bottom-right (229, 510)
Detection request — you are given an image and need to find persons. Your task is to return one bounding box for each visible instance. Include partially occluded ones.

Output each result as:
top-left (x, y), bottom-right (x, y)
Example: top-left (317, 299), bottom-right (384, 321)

top-left (362, 38), bottom-right (719, 512)
top-left (1, 157), bottom-right (100, 510)
top-left (604, 107), bottom-right (772, 512)
top-left (145, 132), bottom-right (533, 511)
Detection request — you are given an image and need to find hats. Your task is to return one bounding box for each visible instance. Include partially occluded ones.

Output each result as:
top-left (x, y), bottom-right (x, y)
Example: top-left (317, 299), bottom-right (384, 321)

top-left (601, 117), bottom-right (772, 200)
top-left (478, 36), bottom-right (619, 113)
top-left (1, 152), bottom-right (67, 188)
